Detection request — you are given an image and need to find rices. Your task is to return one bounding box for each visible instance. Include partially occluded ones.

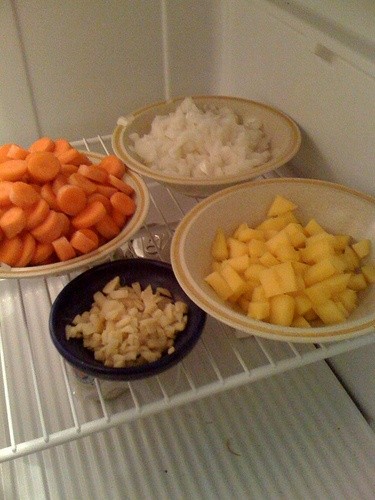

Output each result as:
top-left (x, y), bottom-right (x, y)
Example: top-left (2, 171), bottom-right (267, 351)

top-left (123, 98), bottom-right (272, 175)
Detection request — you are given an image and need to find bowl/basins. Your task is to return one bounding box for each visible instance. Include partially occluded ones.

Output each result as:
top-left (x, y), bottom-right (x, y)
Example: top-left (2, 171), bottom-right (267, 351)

top-left (171, 177), bottom-right (375, 343)
top-left (112, 96), bottom-right (301, 198)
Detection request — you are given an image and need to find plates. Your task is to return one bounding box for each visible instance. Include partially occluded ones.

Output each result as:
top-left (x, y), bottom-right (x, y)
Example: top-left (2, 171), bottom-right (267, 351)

top-left (1, 148), bottom-right (151, 277)
top-left (48, 260), bottom-right (206, 379)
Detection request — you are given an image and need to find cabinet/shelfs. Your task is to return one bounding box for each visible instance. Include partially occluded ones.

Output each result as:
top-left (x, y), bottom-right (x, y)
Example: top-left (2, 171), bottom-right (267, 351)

top-left (0, 134), bottom-right (375, 462)
